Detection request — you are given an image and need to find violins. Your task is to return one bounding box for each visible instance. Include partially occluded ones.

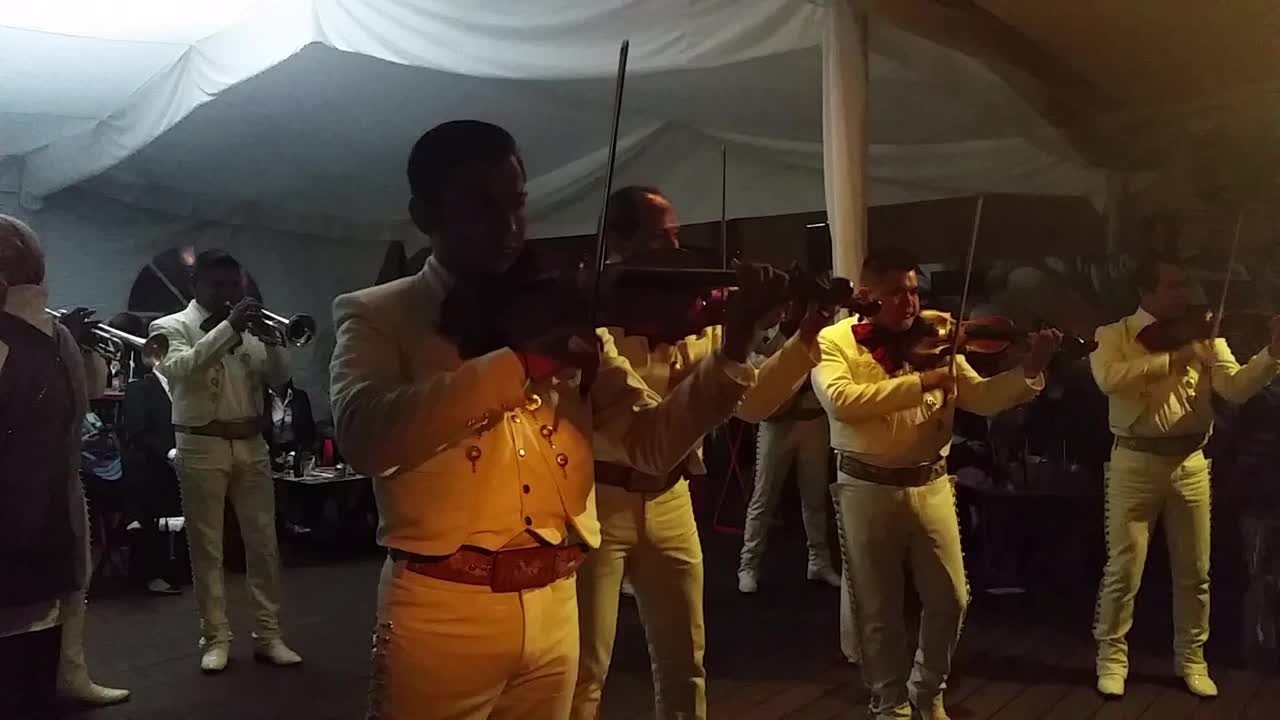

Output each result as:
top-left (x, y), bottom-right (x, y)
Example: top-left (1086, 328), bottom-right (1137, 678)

top-left (1135, 304), bottom-right (1280, 353)
top-left (897, 309), bottom-right (1101, 370)
top-left (840, 288), bottom-right (885, 324)
top-left (618, 247), bottom-right (855, 346)
top-left (434, 243), bottom-right (793, 350)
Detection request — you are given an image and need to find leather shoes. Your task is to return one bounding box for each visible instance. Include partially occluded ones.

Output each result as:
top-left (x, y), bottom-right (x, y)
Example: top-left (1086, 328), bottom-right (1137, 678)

top-left (59, 677), bottom-right (131, 704)
top-left (255, 636), bottom-right (303, 664)
top-left (201, 640), bottom-right (232, 671)
top-left (1183, 675), bottom-right (1218, 697)
top-left (1099, 674), bottom-right (1124, 696)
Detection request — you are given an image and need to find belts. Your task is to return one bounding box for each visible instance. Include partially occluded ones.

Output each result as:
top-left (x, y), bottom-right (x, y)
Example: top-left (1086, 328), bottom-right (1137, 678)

top-left (593, 460), bottom-right (687, 495)
top-left (386, 540), bottom-right (592, 593)
top-left (1114, 435), bottom-right (1197, 458)
top-left (838, 453), bottom-right (948, 487)
top-left (175, 421), bottom-right (265, 439)
top-left (765, 407), bottom-right (829, 424)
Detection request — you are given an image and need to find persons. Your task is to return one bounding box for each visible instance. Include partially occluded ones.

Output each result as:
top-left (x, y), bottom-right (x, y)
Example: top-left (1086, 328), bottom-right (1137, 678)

top-left (737, 240), bottom-right (842, 593)
top-left (1089, 248), bottom-right (1280, 696)
top-left (810, 245), bottom-right (1061, 720)
top-left (124, 373), bottom-right (315, 594)
top-left (327, 120), bottom-right (792, 720)
top-left (0, 215), bottom-right (133, 706)
top-left (569, 185), bottom-right (844, 720)
top-left (148, 250), bottom-right (303, 670)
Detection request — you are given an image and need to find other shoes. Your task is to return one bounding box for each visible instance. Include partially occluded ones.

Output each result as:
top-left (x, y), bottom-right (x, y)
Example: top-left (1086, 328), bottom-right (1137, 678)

top-left (147, 574), bottom-right (188, 594)
top-left (807, 568), bottom-right (843, 585)
top-left (738, 568), bottom-right (757, 592)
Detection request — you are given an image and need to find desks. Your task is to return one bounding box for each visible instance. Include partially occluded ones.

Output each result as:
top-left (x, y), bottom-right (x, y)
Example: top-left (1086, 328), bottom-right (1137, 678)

top-left (274, 467), bottom-right (374, 566)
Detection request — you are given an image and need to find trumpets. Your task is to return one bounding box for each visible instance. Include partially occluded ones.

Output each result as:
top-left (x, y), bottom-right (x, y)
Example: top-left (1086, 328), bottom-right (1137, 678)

top-left (45, 306), bottom-right (170, 368)
top-left (224, 299), bottom-right (319, 352)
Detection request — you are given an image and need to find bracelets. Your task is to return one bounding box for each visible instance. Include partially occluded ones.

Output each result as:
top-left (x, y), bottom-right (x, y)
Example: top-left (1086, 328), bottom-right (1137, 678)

top-left (522, 351), bottom-right (528, 378)
